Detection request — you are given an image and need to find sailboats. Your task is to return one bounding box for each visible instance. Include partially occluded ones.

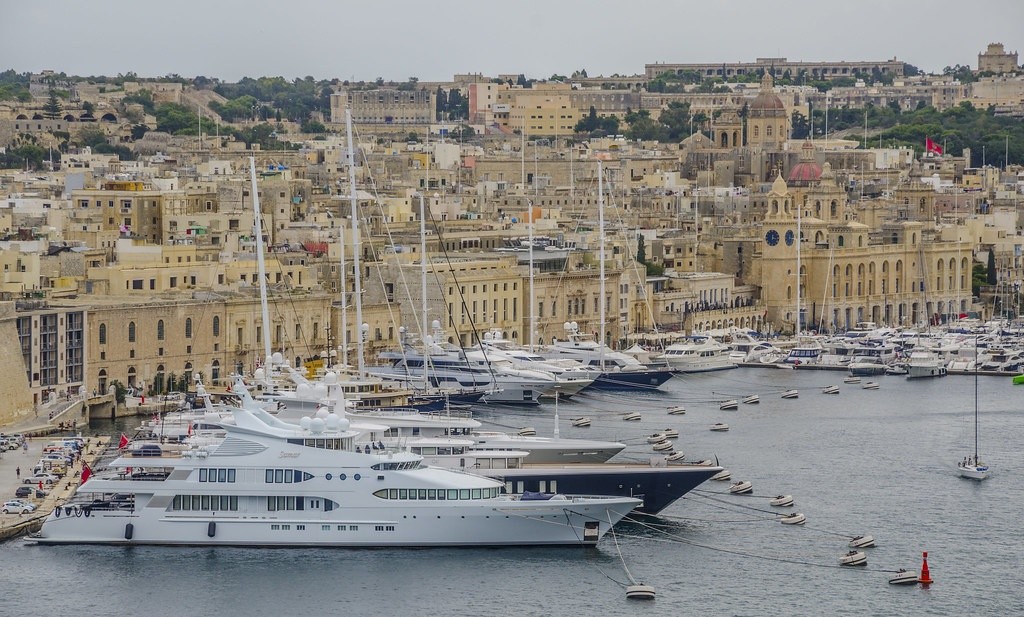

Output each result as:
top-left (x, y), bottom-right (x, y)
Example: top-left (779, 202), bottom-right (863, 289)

top-left (19, 103), bottom-right (1024, 546)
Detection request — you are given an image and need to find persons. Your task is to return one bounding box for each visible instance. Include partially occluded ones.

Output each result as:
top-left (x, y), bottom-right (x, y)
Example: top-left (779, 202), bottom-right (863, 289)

top-left (29, 432), bottom-right (32, 440)
top-left (51, 411), bottom-right (54, 418)
top-left (16, 466), bottom-right (20, 478)
top-left (67, 419), bottom-right (77, 430)
top-left (93, 388), bottom-right (97, 397)
top-left (67, 391), bottom-right (71, 401)
top-left (23, 442), bottom-right (28, 454)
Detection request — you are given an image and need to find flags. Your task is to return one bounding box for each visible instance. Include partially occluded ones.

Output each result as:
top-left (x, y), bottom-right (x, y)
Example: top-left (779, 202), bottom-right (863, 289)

top-left (118, 433), bottom-right (129, 450)
top-left (81, 460), bottom-right (91, 485)
top-left (926, 136), bottom-right (943, 155)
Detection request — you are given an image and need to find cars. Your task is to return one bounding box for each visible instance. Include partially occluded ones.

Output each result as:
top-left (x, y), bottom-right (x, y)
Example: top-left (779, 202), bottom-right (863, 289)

top-left (15, 486), bottom-right (46, 498)
top-left (2, 501), bottom-right (33, 514)
top-left (22, 473), bottom-right (60, 484)
top-left (9, 499), bottom-right (37, 510)
top-left (0, 433), bottom-right (24, 451)
